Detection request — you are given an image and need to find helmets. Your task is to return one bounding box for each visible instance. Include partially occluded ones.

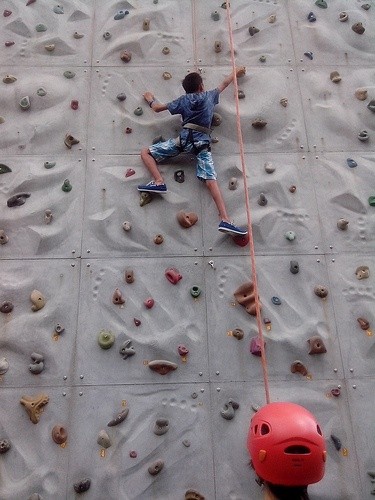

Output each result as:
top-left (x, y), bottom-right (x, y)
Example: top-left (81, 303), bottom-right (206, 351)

top-left (247, 402), bottom-right (327, 487)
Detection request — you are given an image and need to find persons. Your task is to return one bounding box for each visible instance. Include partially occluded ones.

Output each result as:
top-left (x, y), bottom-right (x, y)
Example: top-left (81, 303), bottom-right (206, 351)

top-left (248, 402), bottom-right (327, 500)
top-left (137, 65), bottom-right (248, 236)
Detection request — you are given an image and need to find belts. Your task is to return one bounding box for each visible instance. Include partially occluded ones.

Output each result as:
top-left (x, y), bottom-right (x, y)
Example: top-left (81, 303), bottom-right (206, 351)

top-left (184, 122), bottom-right (210, 133)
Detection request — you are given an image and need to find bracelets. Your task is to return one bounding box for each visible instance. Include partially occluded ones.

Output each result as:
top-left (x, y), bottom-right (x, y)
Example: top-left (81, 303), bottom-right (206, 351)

top-left (148, 100), bottom-right (155, 107)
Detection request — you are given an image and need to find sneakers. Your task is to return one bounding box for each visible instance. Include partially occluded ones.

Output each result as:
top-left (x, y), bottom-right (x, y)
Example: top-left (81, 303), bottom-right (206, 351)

top-left (136, 180), bottom-right (168, 194)
top-left (218, 219), bottom-right (248, 237)
